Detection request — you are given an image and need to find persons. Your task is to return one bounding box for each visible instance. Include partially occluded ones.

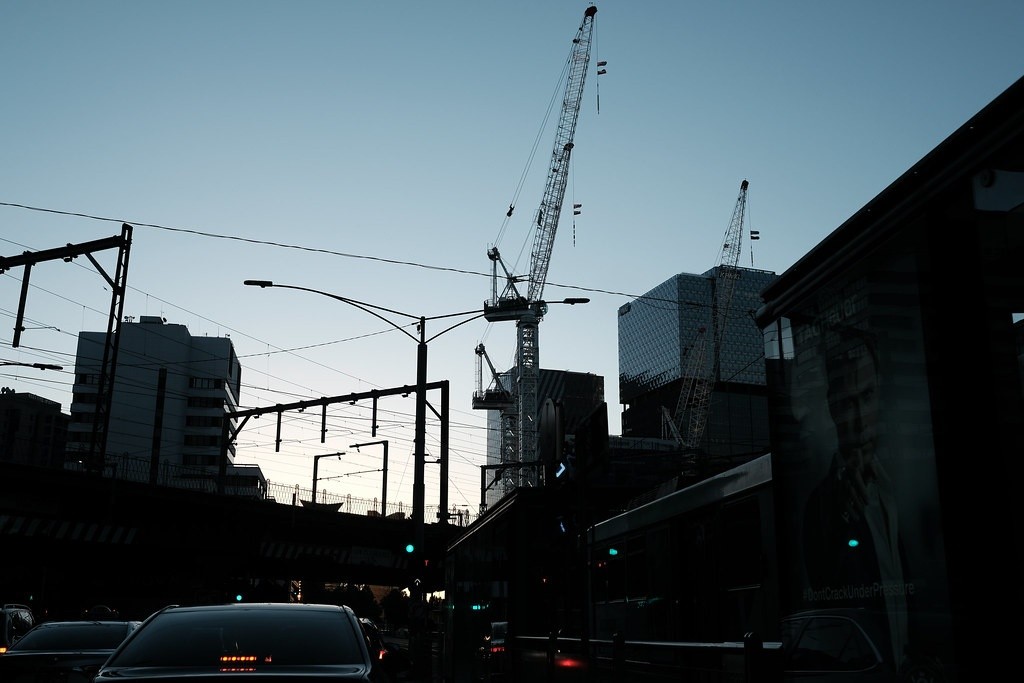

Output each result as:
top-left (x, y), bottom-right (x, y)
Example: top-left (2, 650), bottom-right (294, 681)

top-left (800, 335), bottom-right (916, 673)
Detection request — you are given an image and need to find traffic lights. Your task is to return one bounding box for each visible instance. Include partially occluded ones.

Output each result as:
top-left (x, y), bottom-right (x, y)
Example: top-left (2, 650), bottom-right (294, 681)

top-left (402, 540), bottom-right (418, 562)
top-left (608, 546), bottom-right (622, 562)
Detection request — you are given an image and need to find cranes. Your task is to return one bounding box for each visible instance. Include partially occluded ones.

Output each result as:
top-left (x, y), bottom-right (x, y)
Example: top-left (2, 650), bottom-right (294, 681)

top-left (663, 180), bottom-right (750, 496)
top-left (483, 5), bottom-right (606, 494)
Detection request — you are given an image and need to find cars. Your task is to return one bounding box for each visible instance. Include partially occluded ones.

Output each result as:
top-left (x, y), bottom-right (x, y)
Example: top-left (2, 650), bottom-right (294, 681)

top-left (359, 618), bottom-right (393, 682)
top-left (721, 606), bottom-right (1024, 682)
top-left (0, 620), bottom-right (143, 683)
top-left (90, 603), bottom-right (378, 683)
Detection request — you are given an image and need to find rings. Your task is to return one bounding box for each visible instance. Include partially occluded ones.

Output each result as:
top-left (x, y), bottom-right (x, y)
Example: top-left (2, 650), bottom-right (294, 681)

top-left (837, 467), bottom-right (846, 480)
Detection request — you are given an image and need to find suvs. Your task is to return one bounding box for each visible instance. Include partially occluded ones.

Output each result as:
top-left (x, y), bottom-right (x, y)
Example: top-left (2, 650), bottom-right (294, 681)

top-left (0, 604), bottom-right (38, 651)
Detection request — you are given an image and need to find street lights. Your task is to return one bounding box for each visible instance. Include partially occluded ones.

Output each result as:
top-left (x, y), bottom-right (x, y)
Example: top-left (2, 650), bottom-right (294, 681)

top-left (242, 277), bottom-right (591, 680)
